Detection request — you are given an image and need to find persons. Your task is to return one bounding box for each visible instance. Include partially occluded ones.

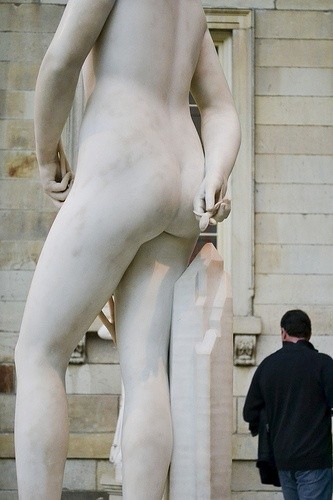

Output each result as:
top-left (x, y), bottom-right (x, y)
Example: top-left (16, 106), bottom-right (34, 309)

top-left (11, 1), bottom-right (241, 499)
top-left (242, 308), bottom-right (333, 500)
